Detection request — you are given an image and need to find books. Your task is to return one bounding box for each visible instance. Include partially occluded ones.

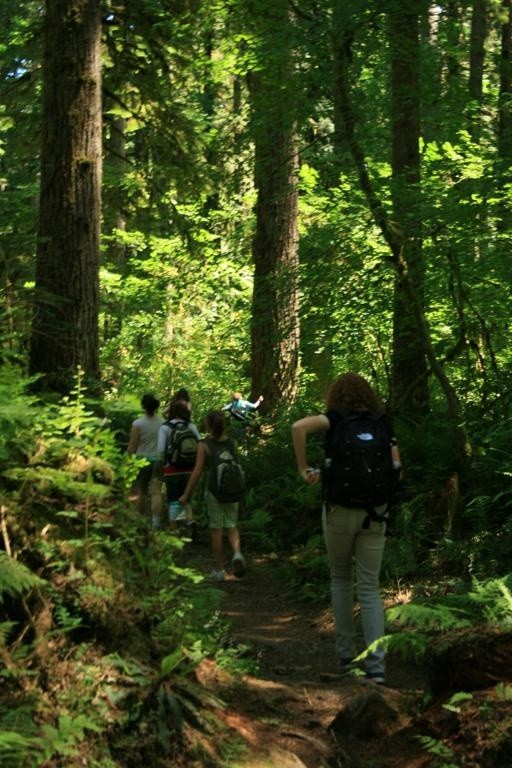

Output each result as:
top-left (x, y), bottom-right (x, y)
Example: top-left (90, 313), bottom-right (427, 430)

top-left (167, 499), bottom-right (194, 523)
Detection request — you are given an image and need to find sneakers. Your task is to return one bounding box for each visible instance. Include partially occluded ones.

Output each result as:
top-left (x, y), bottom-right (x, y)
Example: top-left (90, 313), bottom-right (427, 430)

top-left (232, 552), bottom-right (246, 579)
top-left (319, 668), bottom-right (385, 684)
top-left (207, 569), bottom-right (225, 582)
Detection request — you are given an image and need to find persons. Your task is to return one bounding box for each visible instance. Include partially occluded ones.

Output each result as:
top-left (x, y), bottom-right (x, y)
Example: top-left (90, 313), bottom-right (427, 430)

top-left (176, 409), bottom-right (247, 583)
top-left (126, 393), bottom-right (165, 531)
top-left (169, 388), bottom-right (194, 423)
top-left (290, 372), bottom-right (402, 686)
top-left (220, 391), bottom-right (265, 423)
top-left (156, 397), bottom-right (200, 546)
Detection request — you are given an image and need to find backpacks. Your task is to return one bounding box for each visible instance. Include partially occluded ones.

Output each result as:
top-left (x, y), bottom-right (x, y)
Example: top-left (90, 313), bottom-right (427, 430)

top-left (161, 420), bottom-right (198, 468)
top-left (321, 414), bottom-right (397, 508)
top-left (199, 438), bottom-right (245, 503)
